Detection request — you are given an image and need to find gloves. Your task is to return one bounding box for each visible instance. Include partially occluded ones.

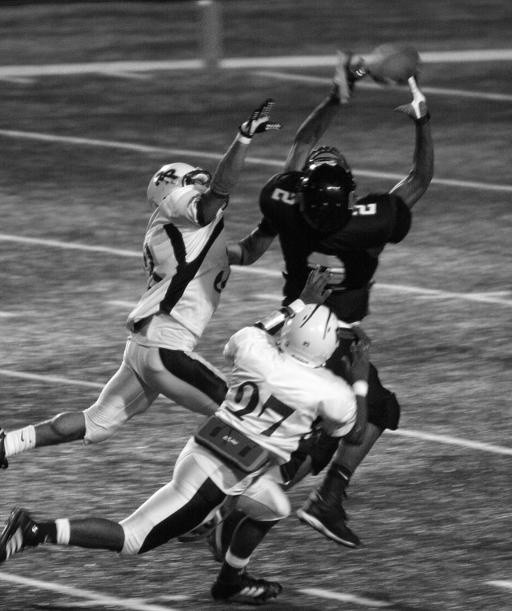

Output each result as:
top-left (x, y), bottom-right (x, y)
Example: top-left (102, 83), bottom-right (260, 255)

top-left (327, 48), bottom-right (368, 106)
top-left (238, 97), bottom-right (284, 145)
top-left (391, 75), bottom-right (433, 129)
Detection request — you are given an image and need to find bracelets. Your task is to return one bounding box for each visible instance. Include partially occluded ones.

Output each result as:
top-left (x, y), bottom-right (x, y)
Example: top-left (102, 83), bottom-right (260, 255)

top-left (351, 377), bottom-right (369, 400)
top-left (289, 298), bottom-right (305, 314)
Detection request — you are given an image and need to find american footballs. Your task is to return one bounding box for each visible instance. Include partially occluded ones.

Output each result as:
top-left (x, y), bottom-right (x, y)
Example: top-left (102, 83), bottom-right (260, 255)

top-left (364, 43), bottom-right (421, 84)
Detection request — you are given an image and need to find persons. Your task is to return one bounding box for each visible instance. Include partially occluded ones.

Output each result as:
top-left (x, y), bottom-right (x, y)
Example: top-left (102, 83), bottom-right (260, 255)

top-left (0, 98), bottom-right (284, 470)
top-left (1, 266), bottom-right (371, 608)
top-left (203, 43), bottom-right (434, 564)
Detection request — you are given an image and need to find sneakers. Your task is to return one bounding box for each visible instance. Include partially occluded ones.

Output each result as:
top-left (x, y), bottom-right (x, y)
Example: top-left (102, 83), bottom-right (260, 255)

top-left (210, 573), bottom-right (283, 607)
top-left (0, 506), bottom-right (41, 564)
top-left (208, 502), bottom-right (249, 562)
top-left (296, 486), bottom-right (361, 549)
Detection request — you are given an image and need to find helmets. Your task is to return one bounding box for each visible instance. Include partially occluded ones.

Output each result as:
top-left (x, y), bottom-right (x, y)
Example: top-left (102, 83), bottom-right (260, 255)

top-left (279, 302), bottom-right (341, 368)
top-left (297, 145), bottom-right (360, 227)
top-left (147, 161), bottom-right (213, 209)
